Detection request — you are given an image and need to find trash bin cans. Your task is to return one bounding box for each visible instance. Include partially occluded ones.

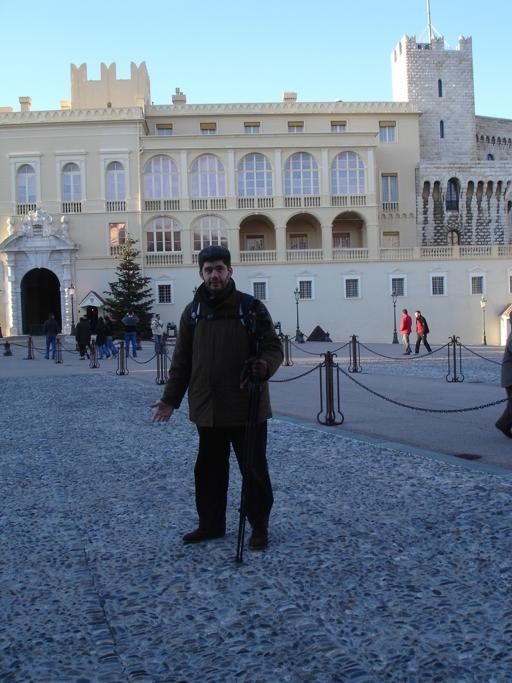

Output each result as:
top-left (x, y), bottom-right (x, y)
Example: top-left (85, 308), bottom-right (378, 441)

top-left (167, 323), bottom-right (176, 337)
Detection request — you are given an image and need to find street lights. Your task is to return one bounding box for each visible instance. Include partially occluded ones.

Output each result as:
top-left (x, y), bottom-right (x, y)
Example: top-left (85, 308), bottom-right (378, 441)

top-left (388, 288), bottom-right (400, 345)
top-left (70, 284), bottom-right (78, 337)
top-left (293, 283), bottom-right (305, 342)
top-left (480, 294), bottom-right (490, 349)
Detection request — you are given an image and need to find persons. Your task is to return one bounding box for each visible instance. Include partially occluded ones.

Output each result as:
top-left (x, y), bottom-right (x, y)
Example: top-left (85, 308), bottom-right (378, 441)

top-left (399, 308), bottom-right (413, 355)
top-left (414, 310), bottom-right (432, 354)
top-left (41, 309), bottom-right (165, 362)
top-left (150, 244), bottom-right (283, 550)
top-left (494, 311), bottom-right (512, 439)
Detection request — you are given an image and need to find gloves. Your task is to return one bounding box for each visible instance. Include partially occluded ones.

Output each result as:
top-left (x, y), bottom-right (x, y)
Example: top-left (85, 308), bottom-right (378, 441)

top-left (239, 356), bottom-right (269, 396)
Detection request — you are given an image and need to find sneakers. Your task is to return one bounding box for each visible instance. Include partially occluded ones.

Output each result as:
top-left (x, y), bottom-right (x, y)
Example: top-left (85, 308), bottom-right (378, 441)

top-left (495, 420), bottom-right (512, 439)
top-left (183, 526), bottom-right (225, 543)
top-left (249, 525), bottom-right (270, 549)
